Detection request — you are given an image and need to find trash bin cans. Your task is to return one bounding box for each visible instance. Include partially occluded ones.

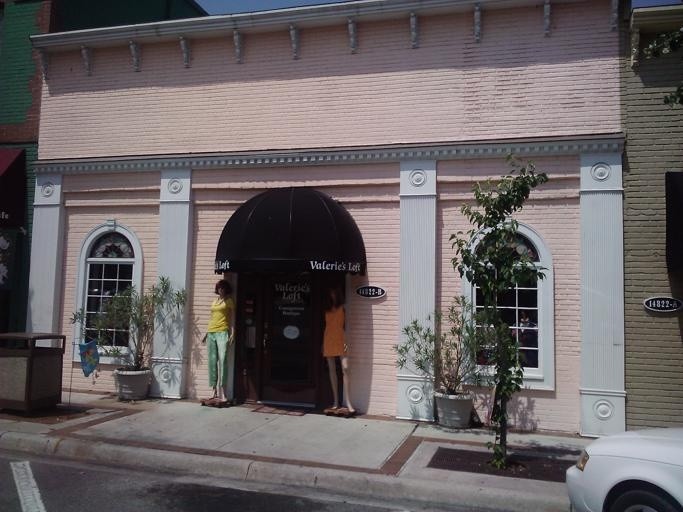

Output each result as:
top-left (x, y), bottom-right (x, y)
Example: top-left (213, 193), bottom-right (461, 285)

top-left (0, 333), bottom-right (66, 417)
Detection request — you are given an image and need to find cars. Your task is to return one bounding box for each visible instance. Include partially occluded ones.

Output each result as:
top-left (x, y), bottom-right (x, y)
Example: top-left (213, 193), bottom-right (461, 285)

top-left (566, 428), bottom-right (683, 512)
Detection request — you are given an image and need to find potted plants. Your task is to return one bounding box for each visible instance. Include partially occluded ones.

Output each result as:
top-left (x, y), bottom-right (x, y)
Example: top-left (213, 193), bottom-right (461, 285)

top-left (66, 274), bottom-right (188, 401)
top-left (388, 296), bottom-right (518, 427)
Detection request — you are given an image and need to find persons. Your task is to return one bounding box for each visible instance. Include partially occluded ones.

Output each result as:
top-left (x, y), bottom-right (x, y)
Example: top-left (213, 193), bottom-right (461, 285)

top-left (323, 283), bottom-right (355, 413)
top-left (200, 279), bottom-right (236, 403)
